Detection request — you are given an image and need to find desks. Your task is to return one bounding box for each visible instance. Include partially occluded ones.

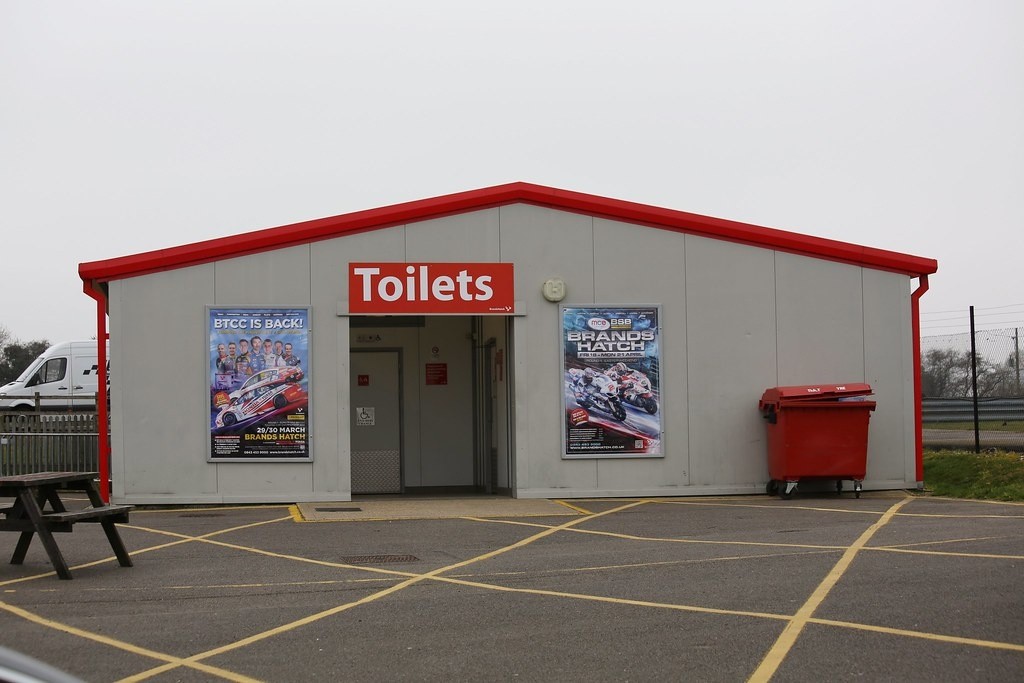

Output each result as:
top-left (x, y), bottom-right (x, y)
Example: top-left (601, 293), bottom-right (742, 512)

top-left (0, 471), bottom-right (133, 580)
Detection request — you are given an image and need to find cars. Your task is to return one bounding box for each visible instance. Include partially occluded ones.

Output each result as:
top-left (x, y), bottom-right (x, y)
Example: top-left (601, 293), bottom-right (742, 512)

top-left (214, 367), bottom-right (308, 429)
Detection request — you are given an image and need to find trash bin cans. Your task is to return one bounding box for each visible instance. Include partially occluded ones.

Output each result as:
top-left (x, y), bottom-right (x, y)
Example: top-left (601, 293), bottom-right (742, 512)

top-left (758, 382), bottom-right (877, 499)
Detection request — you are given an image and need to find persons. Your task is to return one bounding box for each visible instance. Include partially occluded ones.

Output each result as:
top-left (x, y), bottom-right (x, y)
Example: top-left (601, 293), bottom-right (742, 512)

top-left (607, 362), bottom-right (641, 397)
top-left (216, 336), bottom-right (298, 385)
top-left (577, 368), bottom-right (611, 406)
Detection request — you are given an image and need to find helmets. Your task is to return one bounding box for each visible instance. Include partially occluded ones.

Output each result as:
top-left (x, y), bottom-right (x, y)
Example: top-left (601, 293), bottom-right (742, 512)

top-left (581, 367), bottom-right (596, 378)
top-left (615, 362), bottom-right (628, 373)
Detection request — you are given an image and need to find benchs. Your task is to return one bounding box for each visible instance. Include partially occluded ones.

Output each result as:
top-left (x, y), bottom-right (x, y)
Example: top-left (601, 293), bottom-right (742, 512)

top-left (43, 505), bottom-right (136, 532)
top-left (0, 503), bottom-right (15, 531)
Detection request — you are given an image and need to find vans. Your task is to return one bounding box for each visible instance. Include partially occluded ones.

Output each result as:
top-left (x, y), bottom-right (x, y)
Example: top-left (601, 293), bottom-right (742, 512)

top-left (0, 339), bottom-right (110, 432)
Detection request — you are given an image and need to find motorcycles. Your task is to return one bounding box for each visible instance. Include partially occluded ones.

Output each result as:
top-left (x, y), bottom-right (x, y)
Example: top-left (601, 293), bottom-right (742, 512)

top-left (567, 367), bottom-right (658, 422)
